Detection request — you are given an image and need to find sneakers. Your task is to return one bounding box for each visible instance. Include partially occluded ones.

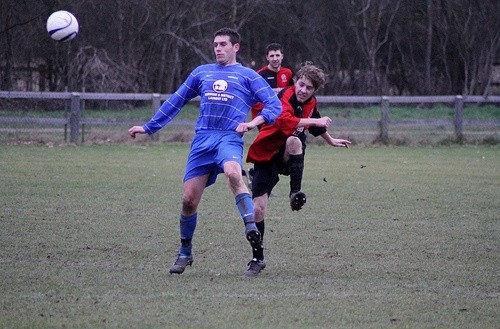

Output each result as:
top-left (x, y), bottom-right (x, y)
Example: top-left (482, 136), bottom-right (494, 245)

top-left (170, 253), bottom-right (194, 273)
top-left (290, 190), bottom-right (306, 211)
top-left (244, 258), bottom-right (266, 276)
top-left (245, 228), bottom-right (264, 252)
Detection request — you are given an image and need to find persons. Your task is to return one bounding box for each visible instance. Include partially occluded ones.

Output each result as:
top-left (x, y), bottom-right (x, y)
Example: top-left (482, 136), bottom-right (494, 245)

top-left (128, 27), bottom-right (282, 275)
top-left (250, 42), bottom-right (297, 132)
top-left (242, 64), bottom-right (353, 251)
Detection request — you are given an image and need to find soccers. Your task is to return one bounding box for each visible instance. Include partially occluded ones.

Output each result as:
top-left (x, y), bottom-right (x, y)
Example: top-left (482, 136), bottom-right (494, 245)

top-left (46, 10), bottom-right (79, 42)
